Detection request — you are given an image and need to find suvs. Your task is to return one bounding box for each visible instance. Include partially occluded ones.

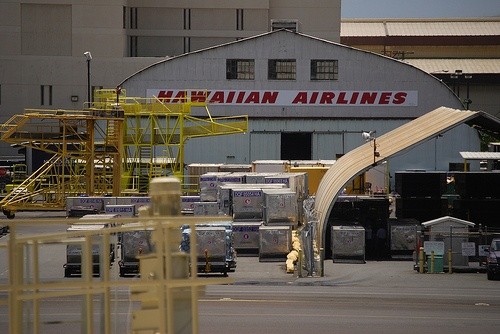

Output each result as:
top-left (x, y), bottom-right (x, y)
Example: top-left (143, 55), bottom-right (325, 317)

top-left (483, 239), bottom-right (500, 281)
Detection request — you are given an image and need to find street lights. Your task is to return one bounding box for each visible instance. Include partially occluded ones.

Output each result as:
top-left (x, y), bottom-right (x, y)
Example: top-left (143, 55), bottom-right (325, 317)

top-left (83, 52), bottom-right (93, 108)
top-left (464, 75), bottom-right (472, 109)
top-left (455, 70), bottom-right (463, 99)
top-left (451, 75), bottom-right (458, 93)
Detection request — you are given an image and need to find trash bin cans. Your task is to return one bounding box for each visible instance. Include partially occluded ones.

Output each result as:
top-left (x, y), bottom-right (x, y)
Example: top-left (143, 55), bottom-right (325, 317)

top-left (427, 256), bottom-right (443, 272)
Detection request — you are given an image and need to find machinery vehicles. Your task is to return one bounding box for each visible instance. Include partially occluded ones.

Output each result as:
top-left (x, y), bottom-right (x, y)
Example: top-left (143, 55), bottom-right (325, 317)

top-left (1, 163), bottom-right (35, 196)
